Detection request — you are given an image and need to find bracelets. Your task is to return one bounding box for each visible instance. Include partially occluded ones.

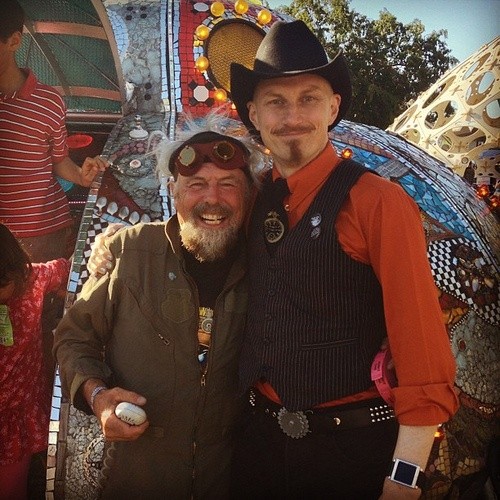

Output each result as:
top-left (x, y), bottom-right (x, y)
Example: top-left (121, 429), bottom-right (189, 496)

top-left (90, 386), bottom-right (107, 413)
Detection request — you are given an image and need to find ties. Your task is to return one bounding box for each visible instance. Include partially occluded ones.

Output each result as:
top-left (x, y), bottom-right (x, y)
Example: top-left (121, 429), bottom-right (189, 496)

top-left (263, 178), bottom-right (289, 256)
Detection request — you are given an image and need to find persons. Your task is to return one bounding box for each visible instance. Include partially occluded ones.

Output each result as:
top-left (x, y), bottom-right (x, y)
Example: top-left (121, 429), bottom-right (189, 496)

top-left (0, 0), bottom-right (111, 263)
top-left (52, 131), bottom-right (255, 499)
top-left (0, 223), bottom-right (71, 499)
top-left (229, 20), bottom-right (459, 500)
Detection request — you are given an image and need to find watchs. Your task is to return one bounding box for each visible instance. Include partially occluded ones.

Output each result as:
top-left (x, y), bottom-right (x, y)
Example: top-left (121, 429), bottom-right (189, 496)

top-left (385, 458), bottom-right (428, 491)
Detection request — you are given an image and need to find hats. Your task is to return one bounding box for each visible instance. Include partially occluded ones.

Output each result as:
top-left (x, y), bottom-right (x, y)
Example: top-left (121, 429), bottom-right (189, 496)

top-left (230, 19), bottom-right (353, 146)
top-left (168, 131), bottom-right (251, 176)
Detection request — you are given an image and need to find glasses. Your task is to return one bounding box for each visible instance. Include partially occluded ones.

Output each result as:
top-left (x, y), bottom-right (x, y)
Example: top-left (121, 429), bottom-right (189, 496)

top-left (175, 140), bottom-right (249, 177)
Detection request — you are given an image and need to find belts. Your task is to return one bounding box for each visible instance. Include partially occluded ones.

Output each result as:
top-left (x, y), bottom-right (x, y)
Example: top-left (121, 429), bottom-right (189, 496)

top-left (248, 388), bottom-right (397, 440)
